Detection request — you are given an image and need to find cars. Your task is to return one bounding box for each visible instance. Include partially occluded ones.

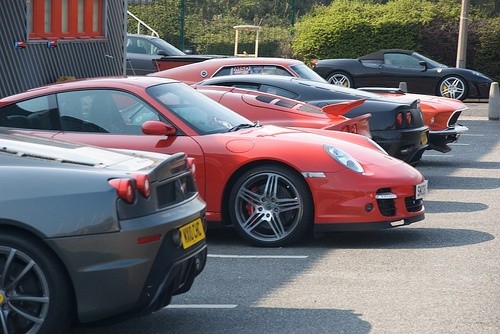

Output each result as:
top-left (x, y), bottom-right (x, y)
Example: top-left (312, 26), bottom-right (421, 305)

top-left (310, 49), bottom-right (493, 102)
top-left (0, 131), bottom-right (208, 334)
top-left (125, 34), bottom-right (188, 77)
top-left (145, 57), bottom-right (469, 153)
top-left (189, 73), bottom-right (430, 169)
top-left (0, 75), bottom-right (429, 248)
top-left (191, 85), bottom-right (373, 140)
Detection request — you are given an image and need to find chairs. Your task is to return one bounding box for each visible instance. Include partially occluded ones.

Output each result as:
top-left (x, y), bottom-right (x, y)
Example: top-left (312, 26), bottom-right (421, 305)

top-left (89, 93), bottom-right (126, 133)
